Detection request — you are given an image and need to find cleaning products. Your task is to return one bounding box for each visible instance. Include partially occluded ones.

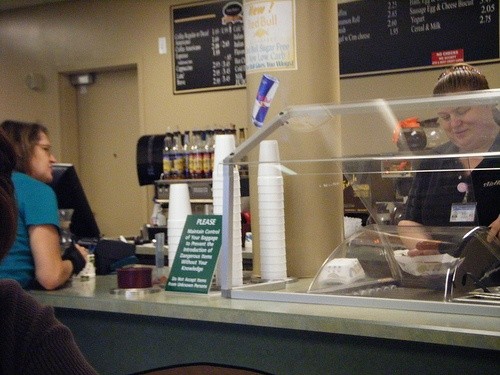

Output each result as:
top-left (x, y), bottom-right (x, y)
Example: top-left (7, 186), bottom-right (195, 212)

top-left (73, 243), bottom-right (97, 296)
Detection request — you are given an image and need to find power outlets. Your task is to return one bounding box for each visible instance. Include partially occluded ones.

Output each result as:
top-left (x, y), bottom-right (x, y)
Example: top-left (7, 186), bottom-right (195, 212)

top-left (154, 175), bottom-right (250, 225)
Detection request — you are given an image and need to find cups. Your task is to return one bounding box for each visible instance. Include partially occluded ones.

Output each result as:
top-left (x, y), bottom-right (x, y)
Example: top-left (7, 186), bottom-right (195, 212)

top-left (167, 183), bottom-right (193, 271)
top-left (256, 140), bottom-right (287, 281)
top-left (212, 134), bottom-right (244, 289)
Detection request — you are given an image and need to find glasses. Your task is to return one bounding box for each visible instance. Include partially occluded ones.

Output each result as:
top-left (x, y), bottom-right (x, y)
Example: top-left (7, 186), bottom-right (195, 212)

top-left (31, 143), bottom-right (51, 156)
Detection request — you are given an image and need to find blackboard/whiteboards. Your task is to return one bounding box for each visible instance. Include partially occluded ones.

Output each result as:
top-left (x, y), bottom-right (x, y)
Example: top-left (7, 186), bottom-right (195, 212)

top-left (170, 0), bottom-right (500, 94)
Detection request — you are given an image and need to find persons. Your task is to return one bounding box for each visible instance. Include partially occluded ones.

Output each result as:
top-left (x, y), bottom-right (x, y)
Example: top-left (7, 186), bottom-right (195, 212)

top-left (397, 63), bottom-right (500, 255)
top-left (0, 175), bottom-right (101, 375)
top-left (0, 120), bottom-right (88, 291)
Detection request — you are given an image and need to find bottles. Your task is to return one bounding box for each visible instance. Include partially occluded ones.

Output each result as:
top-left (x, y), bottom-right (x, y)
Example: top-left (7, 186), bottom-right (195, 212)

top-left (59, 208), bottom-right (74, 249)
top-left (162, 128), bottom-right (245, 178)
top-left (152, 202), bottom-right (163, 225)
top-left (396, 127), bottom-right (427, 151)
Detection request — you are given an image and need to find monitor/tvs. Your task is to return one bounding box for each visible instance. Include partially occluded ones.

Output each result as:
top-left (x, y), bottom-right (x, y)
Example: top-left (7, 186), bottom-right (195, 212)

top-left (48, 163), bottom-right (100, 238)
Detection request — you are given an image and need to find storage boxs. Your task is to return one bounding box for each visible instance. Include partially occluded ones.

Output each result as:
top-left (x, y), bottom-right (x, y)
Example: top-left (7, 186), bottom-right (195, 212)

top-left (396, 254), bottom-right (466, 276)
top-left (319, 257), bottom-right (364, 283)
